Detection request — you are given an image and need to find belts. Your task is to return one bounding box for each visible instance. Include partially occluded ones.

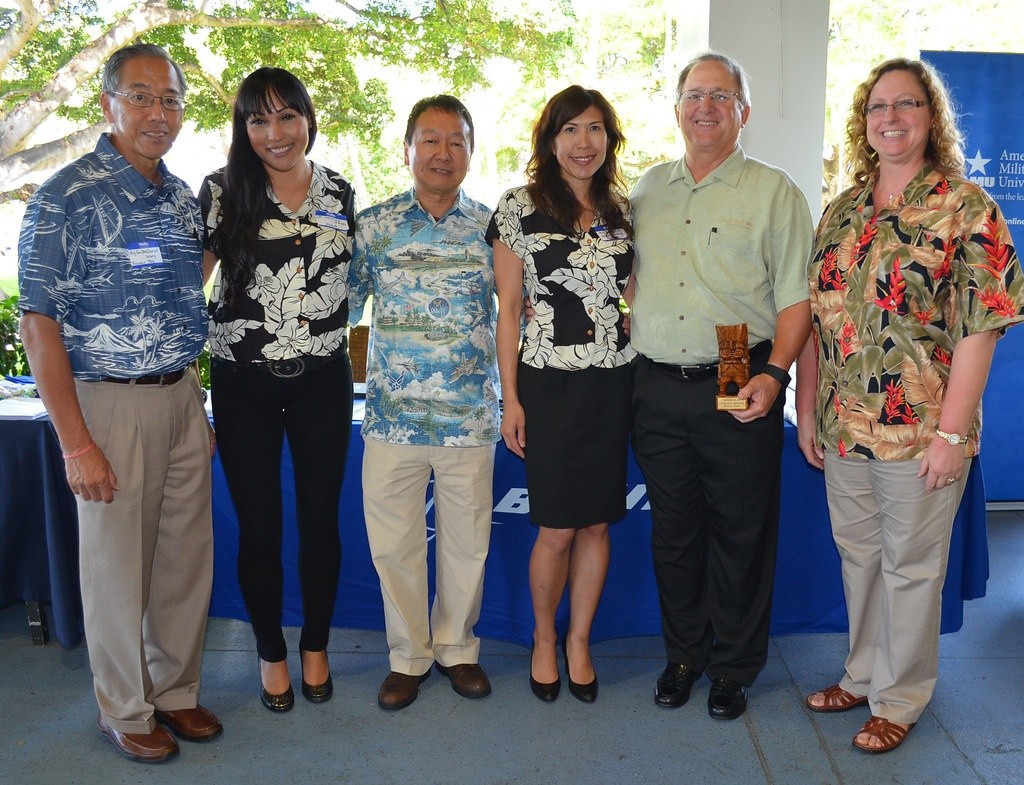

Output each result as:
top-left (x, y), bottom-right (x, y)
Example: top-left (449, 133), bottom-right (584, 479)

top-left (104, 366), bottom-right (189, 385)
top-left (220, 348), bottom-right (350, 378)
top-left (650, 340), bottom-right (770, 383)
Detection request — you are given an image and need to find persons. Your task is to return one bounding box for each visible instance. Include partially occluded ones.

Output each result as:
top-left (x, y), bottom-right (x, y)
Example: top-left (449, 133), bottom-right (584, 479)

top-left (19, 44), bottom-right (225, 761)
top-left (787, 51), bottom-right (1023, 755)
top-left (196, 68), bottom-right (353, 714)
top-left (484, 82), bottom-right (636, 704)
top-left (347, 95), bottom-right (628, 711)
top-left (520, 51), bottom-right (813, 717)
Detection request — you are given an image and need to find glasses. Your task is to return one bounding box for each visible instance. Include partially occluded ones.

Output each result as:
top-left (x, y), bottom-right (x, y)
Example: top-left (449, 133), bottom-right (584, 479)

top-left (864, 98), bottom-right (930, 117)
top-left (110, 90), bottom-right (188, 111)
top-left (680, 90), bottom-right (742, 103)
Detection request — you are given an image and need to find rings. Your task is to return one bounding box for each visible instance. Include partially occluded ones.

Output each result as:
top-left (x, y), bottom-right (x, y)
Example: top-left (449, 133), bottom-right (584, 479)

top-left (947, 477), bottom-right (955, 484)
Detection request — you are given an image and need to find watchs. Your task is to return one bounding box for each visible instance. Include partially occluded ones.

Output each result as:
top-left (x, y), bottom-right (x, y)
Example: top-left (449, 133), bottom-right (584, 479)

top-left (936, 429), bottom-right (968, 446)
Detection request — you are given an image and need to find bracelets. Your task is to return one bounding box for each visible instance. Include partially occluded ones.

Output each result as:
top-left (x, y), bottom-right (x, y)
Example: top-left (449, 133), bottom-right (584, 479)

top-left (63, 440), bottom-right (95, 460)
top-left (761, 364), bottom-right (791, 389)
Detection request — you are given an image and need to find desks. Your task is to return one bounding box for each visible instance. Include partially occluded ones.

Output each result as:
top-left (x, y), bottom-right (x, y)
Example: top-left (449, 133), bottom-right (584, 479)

top-left (0, 382), bottom-right (991, 650)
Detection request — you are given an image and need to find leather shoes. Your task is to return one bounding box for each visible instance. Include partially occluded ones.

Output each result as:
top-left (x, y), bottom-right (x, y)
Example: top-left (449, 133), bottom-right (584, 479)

top-left (378, 670), bottom-right (431, 709)
top-left (97, 710), bottom-right (180, 764)
top-left (156, 704), bottom-right (222, 743)
top-left (301, 648), bottom-right (333, 702)
top-left (655, 665), bottom-right (698, 708)
top-left (434, 659), bottom-right (492, 698)
top-left (259, 662), bottom-right (295, 712)
top-left (707, 678), bottom-right (748, 720)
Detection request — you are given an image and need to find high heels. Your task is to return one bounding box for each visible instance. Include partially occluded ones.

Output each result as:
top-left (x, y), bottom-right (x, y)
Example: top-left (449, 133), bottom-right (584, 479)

top-left (564, 637), bottom-right (598, 702)
top-left (530, 638), bottom-right (561, 701)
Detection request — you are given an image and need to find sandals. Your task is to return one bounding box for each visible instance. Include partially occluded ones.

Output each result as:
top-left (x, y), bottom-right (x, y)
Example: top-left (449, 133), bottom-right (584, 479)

top-left (852, 716), bottom-right (918, 753)
top-left (807, 685), bottom-right (869, 710)
